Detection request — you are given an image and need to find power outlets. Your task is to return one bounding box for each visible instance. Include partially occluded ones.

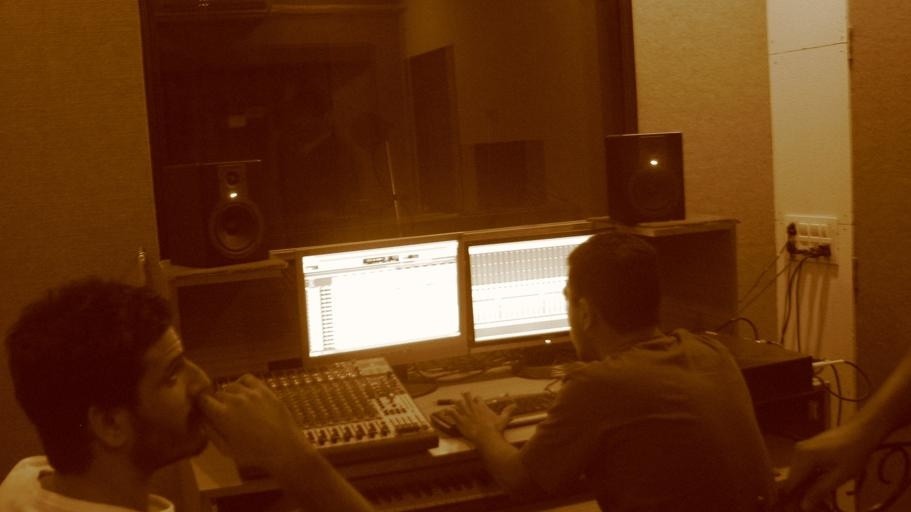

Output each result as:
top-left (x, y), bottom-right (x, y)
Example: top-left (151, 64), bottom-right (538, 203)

top-left (784, 216), bottom-right (839, 265)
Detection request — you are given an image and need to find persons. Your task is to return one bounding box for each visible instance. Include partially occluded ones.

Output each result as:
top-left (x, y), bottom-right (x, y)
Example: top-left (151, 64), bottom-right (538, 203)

top-left (784, 341), bottom-right (909, 508)
top-left (441, 227), bottom-right (785, 508)
top-left (0, 274), bottom-right (380, 511)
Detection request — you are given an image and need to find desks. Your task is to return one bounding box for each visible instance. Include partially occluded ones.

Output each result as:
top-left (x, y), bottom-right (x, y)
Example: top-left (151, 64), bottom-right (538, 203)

top-left (186, 339), bottom-right (814, 511)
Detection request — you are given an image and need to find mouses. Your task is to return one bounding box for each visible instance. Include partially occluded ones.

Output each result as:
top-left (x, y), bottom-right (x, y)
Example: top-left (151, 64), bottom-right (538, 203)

top-left (561, 373), bottom-right (574, 384)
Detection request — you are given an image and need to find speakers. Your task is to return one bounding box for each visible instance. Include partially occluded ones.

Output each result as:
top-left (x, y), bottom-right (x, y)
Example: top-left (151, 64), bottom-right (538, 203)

top-left (605, 130), bottom-right (686, 228)
top-left (156, 157), bottom-right (270, 268)
top-left (476, 139), bottom-right (547, 209)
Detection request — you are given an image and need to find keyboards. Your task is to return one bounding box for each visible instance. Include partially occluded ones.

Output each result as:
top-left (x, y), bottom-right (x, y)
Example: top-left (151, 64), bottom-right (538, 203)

top-left (431, 390), bottom-right (564, 435)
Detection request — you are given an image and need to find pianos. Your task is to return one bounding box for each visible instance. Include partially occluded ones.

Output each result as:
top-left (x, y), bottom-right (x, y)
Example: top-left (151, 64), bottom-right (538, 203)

top-left (359, 472), bottom-right (507, 512)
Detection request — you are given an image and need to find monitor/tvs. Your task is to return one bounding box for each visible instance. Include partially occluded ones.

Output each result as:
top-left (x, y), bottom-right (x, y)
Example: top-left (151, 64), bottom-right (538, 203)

top-left (293, 230), bottom-right (469, 398)
top-left (462, 220), bottom-right (595, 379)
